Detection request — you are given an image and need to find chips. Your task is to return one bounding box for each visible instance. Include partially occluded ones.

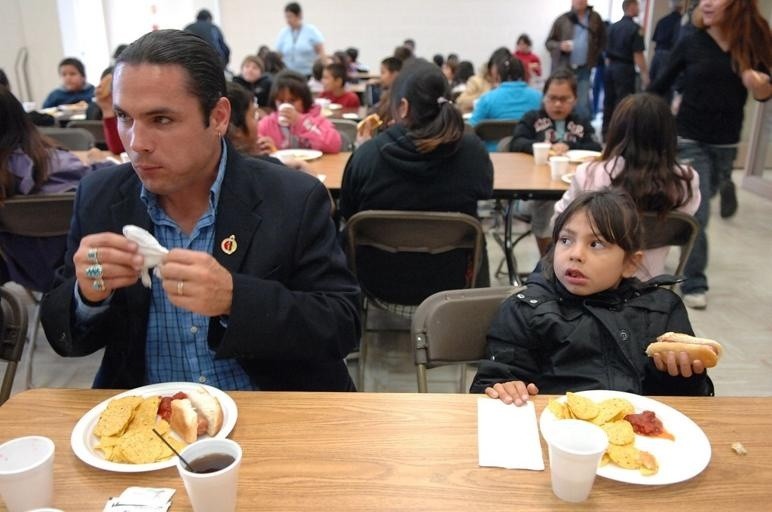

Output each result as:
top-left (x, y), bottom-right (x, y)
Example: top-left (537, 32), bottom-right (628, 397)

top-left (548, 391), bottom-right (659, 476)
top-left (93, 396), bottom-right (187, 465)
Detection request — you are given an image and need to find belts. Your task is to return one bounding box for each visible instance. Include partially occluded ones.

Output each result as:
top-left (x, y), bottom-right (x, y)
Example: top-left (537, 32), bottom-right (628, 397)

top-left (568, 63), bottom-right (588, 71)
top-left (608, 58), bottom-right (632, 65)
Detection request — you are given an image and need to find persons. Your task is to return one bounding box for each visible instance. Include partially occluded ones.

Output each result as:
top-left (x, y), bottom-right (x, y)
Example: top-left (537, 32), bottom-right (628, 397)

top-left (465, 184), bottom-right (717, 405)
top-left (549, 88), bottom-right (703, 292)
top-left (87, 265), bottom-right (103, 279)
top-left (652, 0), bottom-right (685, 52)
top-left (643, 0), bottom-right (772, 312)
top-left (93, 279), bottom-right (106, 293)
top-left (0, 1), bottom-right (611, 323)
top-left (89, 249), bottom-right (97, 262)
top-left (602, 1), bottom-right (652, 140)
top-left (37, 29), bottom-right (368, 391)
top-left (177, 280), bottom-right (184, 296)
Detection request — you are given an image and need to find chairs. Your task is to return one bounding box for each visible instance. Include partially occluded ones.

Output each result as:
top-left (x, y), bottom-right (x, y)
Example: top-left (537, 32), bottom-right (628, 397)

top-left (1, 192), bottom-right (76, 390)
top-left (345, 209), bottom-right (484, 392)
top-left (1, 287), bottom-right (30, 407)
top-left (481, 134), bottom-right (541, 288)
top-left (634, 206), bottom-right (697, 293)
top-left (411, 284), bottom-right (528, 393)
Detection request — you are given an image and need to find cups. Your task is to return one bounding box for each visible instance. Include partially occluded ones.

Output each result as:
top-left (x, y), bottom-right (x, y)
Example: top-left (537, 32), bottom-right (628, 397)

top-left (175, 437), bottom-right (242, 511)
top-left (277, 104), bottom-right (294, 128)
top-left (1, 435), bottom-right (57, 511)
top-left (549, 157), bottom-right (570, 180)
top-left (548, 418), bottom-right (609, 503)
top-left (532, 142), bottom-right (550, 167)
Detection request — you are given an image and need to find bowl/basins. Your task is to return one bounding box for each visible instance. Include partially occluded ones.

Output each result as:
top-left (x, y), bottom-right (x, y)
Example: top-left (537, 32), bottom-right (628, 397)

top-left (23, 102), bottom-right (87, 117)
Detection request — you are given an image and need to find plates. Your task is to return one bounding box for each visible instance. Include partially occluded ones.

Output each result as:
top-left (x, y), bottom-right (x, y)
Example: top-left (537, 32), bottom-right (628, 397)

top-left (559, 174), bottom-right (574, 182)
top-left (68, 382), bottom-right (239, 476)
top-left (272, 150), bottom-right (323, 163)
top-left (564, 150), bottom-right (601, 164)
top-left (539, 387), bottom-right (711, 486)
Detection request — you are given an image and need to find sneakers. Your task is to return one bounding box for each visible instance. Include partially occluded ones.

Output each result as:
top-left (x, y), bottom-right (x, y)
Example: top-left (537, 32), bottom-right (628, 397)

top-left (681, 289), bottom-right (708, 309)
top-left (721, 182), bottom-right (737, 219)
top-left (536, 235), bottom-right (555, 258)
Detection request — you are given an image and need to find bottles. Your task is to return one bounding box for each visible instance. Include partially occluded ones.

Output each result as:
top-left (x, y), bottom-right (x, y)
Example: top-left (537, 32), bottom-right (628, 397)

top-left (318, 99), bottom-right (344, 120)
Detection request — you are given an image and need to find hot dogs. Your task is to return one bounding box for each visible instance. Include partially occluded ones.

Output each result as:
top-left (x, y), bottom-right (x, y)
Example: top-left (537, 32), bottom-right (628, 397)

top-left (170, 385), bottom-right (223, 443)
top-left (646, 331), bottom-right (722, 368)
